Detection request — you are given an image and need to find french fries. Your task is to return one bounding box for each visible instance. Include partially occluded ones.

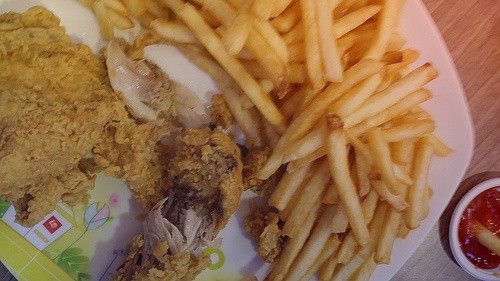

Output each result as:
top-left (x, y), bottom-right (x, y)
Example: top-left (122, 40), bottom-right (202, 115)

top-left (81, 1), bottom-right (456, 280)
top-left (474, 220), bottom-right (500, 257)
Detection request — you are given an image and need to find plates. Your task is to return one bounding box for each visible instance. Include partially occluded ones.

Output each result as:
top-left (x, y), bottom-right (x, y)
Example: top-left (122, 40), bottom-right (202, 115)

top-left (448, 177), bottom-right (500, 280)
top-left (0, 1), bottom-right (475, 280)
